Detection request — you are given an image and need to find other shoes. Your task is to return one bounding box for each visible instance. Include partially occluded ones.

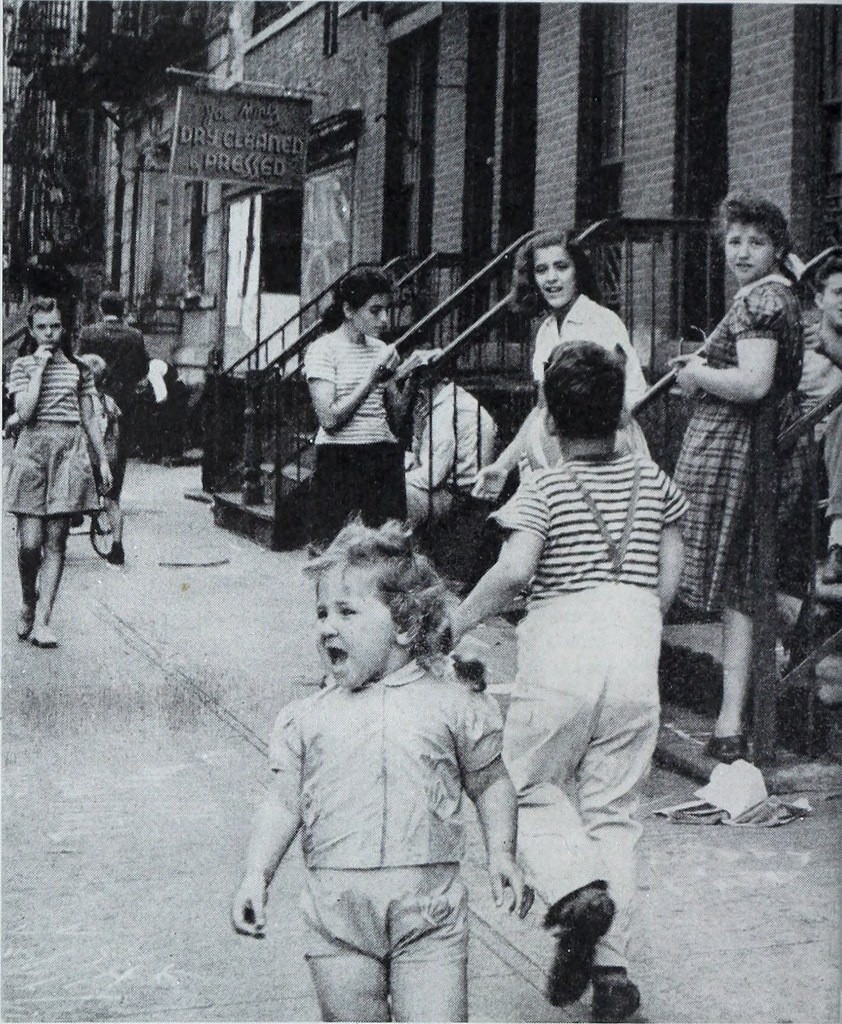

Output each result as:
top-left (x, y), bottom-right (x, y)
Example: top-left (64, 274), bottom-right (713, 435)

top-left (704, 728), bottom-right (749, 767)
top-left (105, 540), bottom-right (124, 565)
top-left (781, 600), bottom-right (832, 677)
top-left (545, 883), bottom-right (642, 1023)
top-left (14, 599), bottom-right (59, 646)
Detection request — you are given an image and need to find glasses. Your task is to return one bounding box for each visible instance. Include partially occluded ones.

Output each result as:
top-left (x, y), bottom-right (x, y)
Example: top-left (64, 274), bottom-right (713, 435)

top-left (672, 324), bottom-right (711, 379)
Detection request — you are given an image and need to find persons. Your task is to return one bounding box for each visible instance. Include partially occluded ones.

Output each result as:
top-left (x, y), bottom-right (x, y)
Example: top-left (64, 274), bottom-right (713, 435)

top-left (438, 341), bottom-right (691, 1024)
top-left (76, 291), bottom-right (151, 563)
top-left (799, 248), bottom-right (841, 581)
top-left (405, 368), bottom-right (500, 530)
top-left (5, 297), bottom-right (101, 647)
top-left (305, 270), bottom-right (429, 561)
top-left (471, 231), bottom-right (651, 500)
top-left (2, 353), bottom-right (123, 470)
top-left (668, 190), bottom-right (817, 763)
top-left (232, 524), bottom-right (535, 1022)
